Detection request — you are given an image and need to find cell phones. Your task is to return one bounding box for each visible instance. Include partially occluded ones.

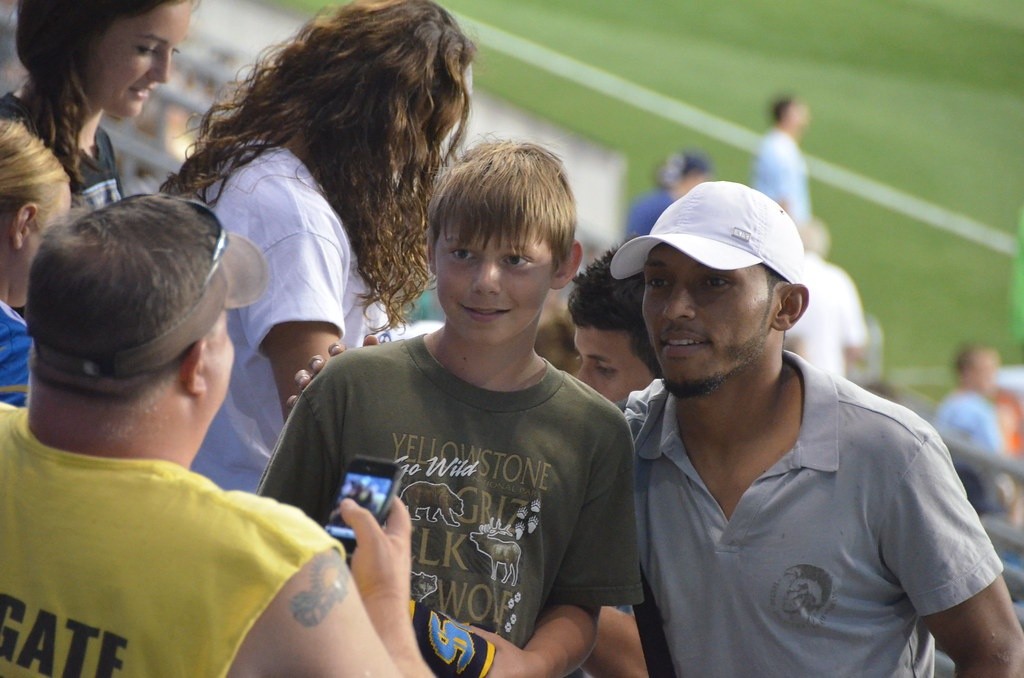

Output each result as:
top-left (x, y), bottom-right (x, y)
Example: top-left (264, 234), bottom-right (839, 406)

top-left (322, 456), bottom-right (401, 561)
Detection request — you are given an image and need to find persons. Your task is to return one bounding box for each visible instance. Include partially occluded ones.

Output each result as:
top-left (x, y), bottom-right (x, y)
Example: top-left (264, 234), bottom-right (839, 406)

top-left (0, 195), bottom-right (440, 678)
top-left (0, 0), bottom-right (1024, 678)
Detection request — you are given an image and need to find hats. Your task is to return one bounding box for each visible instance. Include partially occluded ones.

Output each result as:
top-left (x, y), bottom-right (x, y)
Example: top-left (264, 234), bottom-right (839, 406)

top-left (29, 191), bottom-right (269, 380)
top-left (610, 181), bottom-right (806, 285)
